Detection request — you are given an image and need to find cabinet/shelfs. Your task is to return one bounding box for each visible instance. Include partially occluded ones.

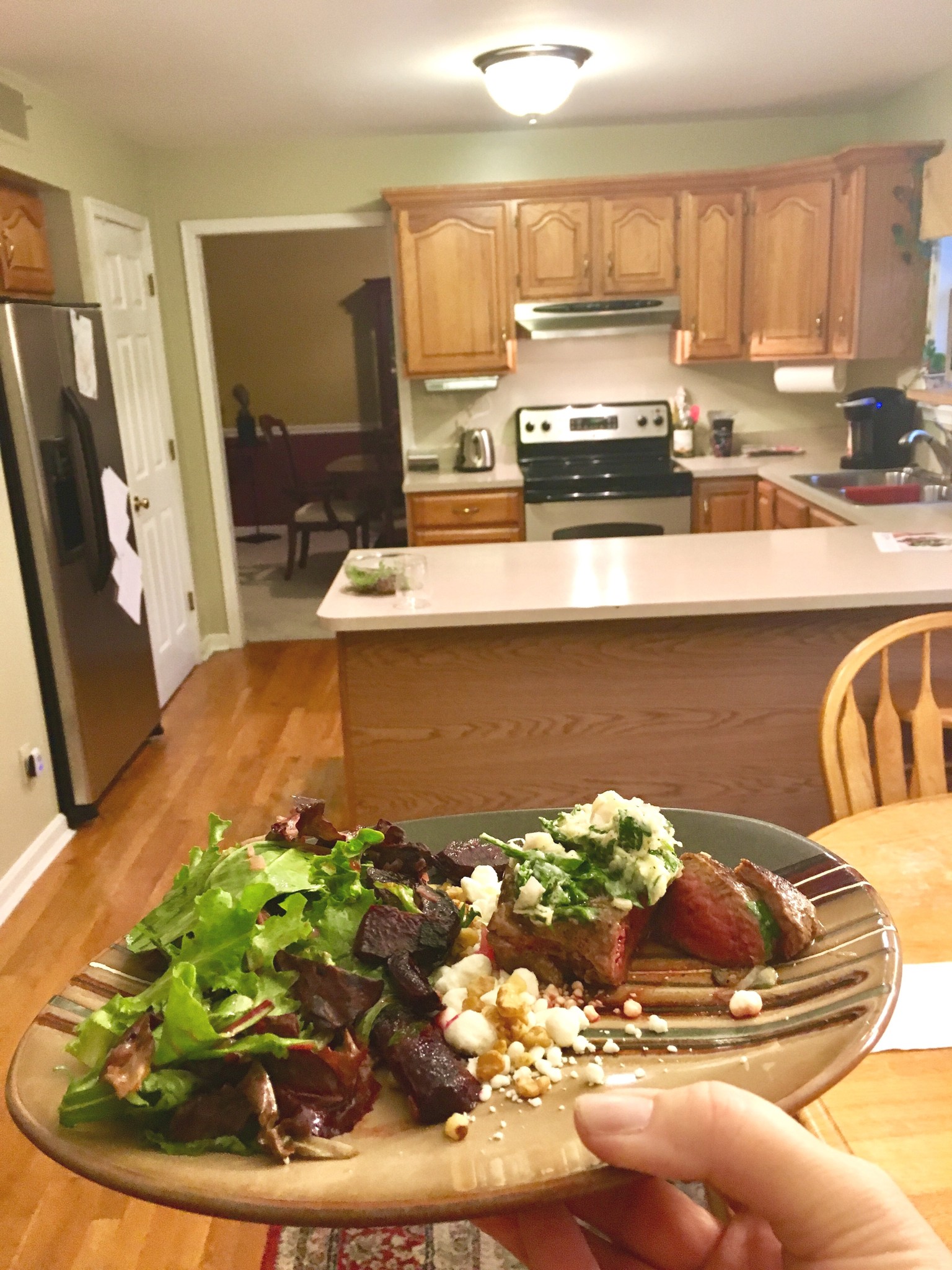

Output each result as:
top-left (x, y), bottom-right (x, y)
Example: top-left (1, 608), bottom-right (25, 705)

top-left (742, 151), bottom-right (831, 361)
top-left (378, 181), bottom-right (519, 380)
top-left (829, 138), bottom-right (944, 362)
top-left (668, 167), bottom-right (748, 366)
top-left (755, 476), bottom-right (856, 531)
top-left (405, 487), bottom-right (526, 547)
top-left (690, 474), bottom-right (758, 533)
top-left (508, 170), bottom-right (680, 305)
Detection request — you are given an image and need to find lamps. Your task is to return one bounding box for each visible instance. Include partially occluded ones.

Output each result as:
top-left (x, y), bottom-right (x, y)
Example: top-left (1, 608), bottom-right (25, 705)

top-left (473, 43), bottom-right (593, 130)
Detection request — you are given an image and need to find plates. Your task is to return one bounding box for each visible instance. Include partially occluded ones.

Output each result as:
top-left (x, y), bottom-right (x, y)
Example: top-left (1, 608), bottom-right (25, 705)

top-left (2, 805), bottom-right (902, 1227)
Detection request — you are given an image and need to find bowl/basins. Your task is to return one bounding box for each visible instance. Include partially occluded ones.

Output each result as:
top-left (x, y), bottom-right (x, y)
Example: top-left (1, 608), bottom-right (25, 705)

top-left (342, 553), bottom-right (428, 595)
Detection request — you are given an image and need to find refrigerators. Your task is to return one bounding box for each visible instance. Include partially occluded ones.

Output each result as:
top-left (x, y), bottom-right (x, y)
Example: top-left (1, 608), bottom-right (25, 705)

top-left (1, 298), bottom-right (161, 832)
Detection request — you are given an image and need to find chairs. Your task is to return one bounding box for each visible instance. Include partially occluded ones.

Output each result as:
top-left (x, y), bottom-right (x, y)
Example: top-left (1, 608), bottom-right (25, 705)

top-left (257, 414), bottom-right (376, 583)
top-left (815, 609), bottom-right (952, 825)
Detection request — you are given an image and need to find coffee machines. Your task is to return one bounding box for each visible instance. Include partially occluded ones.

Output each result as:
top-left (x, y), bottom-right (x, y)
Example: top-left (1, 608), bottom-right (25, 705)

top-left (836, 386), bottom-right (917, 470)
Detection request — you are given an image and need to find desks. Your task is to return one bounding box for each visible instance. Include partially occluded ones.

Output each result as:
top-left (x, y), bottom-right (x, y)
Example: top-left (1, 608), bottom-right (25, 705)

top-left (323, 450), bottom-right (406, 544)
top-left (794, 792), bottom-right (952, 1252)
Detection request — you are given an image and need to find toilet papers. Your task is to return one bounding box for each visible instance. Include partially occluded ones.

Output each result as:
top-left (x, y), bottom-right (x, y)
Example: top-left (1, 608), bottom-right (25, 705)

top-left (772, 365), bottom-right (848, 394)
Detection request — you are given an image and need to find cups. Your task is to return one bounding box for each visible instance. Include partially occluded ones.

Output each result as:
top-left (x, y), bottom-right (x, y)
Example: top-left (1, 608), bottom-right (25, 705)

top-left (673, 429), bottom-right (693, 457)
top-left (396, 560), bottom-right (433, 609)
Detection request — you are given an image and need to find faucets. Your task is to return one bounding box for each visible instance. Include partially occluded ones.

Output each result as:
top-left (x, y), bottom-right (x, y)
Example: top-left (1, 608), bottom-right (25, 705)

top-left (896, 419), bottom-right (952, 481)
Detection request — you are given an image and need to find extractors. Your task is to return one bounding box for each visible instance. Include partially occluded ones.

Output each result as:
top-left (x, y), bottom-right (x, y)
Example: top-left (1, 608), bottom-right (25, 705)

top-left (513, 296), bottom-right (681, 340)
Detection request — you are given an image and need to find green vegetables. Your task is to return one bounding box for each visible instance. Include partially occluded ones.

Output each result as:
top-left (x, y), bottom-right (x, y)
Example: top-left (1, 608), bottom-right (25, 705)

top-left (54, 794), bottom-right (783, 1158)
top-left (347, 560), bottom-right (401, 593)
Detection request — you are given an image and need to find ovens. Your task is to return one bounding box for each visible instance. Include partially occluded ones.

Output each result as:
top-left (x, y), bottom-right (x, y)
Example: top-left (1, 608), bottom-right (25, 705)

top-left (524, 486), bottom-right (690, 541)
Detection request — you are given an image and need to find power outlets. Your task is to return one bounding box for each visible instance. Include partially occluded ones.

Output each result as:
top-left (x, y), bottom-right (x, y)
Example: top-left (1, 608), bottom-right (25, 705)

top-left (18, 744), bottom-right (38, 785)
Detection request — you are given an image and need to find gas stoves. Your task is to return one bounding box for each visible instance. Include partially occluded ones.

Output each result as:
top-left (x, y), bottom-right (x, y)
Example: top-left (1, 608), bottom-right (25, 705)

top-left (515, 401), bottom-right (693, 490)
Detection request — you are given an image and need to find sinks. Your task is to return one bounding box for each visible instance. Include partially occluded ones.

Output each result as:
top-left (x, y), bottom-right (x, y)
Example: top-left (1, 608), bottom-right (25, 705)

top-left (835, 485), bottom-right (952, 505)
top-left (788, 466), bottom-right (945, 495)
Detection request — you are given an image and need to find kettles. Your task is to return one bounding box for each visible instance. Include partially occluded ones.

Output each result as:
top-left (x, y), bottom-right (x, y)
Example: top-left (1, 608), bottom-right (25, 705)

top-left (454, 428), bottom-right (495, 473)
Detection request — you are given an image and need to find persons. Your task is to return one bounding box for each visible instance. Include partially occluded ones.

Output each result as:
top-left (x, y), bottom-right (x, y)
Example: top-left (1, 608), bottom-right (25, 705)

top-left (568, 1080), bottom-right (951, 1270)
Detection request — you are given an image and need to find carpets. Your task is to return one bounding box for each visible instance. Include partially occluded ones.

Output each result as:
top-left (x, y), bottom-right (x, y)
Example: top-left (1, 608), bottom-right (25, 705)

top-left (260, 1178), bottom-right (735, 1270)
